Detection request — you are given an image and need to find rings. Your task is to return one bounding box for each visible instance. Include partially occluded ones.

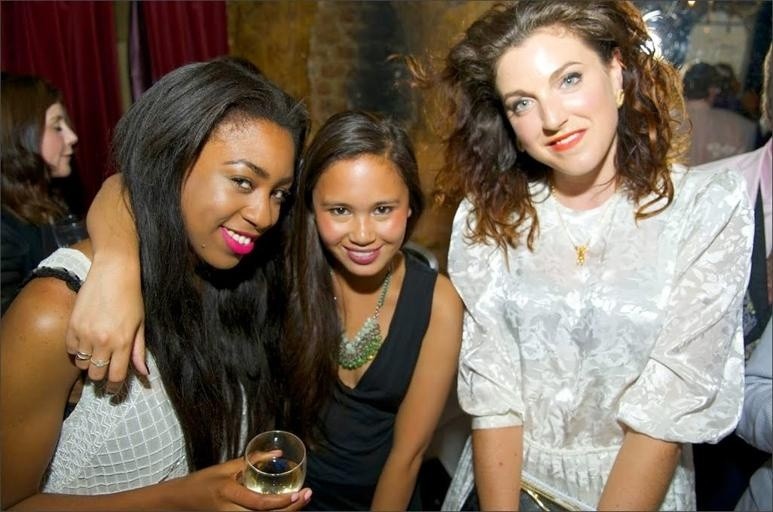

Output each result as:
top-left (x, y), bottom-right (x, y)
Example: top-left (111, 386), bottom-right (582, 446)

top-left (90, 357), bottom-right (109, 367)
top-left (76, 351), bottom-right (92, 360)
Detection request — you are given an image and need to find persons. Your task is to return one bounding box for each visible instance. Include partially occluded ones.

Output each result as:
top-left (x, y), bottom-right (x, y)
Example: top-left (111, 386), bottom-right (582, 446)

top-left (0, 56), bottom-right (311, 512)
top-left (0, 71), bottom-right (78, 320)
top-left (66, 108), bottom-right (464, 512)
top-left (385, 0), bottom-right (755, 512)
top-left (667, 43), bottom-right (772, 512)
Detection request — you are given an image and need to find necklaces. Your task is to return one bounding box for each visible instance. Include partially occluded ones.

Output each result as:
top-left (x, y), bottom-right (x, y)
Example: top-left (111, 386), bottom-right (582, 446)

top-left (329, 260), bottom-right (393, 368)
top-left (548, 176), bottom-right (618, 266)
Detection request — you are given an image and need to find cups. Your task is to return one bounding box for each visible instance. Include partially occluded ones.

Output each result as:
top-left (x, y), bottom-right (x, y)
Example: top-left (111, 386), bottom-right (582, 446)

top-left (245, 429), bottom-right (308, 494)
